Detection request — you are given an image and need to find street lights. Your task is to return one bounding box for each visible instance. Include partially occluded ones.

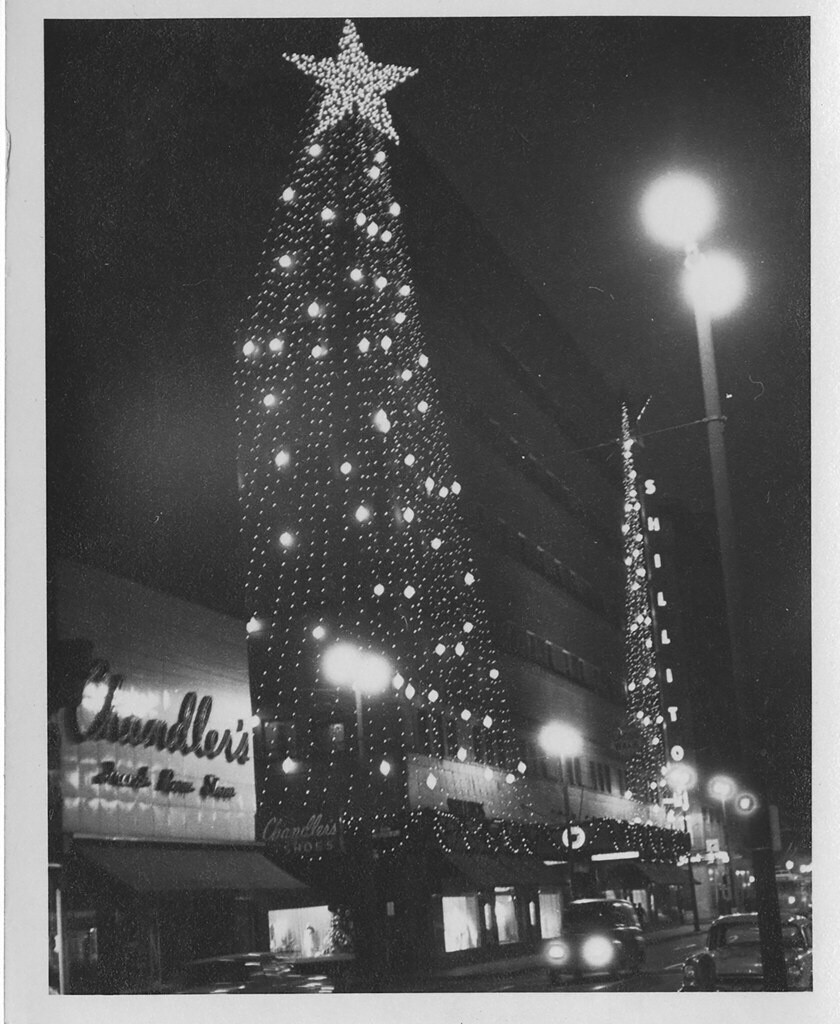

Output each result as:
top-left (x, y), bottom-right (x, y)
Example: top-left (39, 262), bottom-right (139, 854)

top-left (708, 773), bottom-right (745, 913)
top-left (637, 171), bottom-right (794, 992)
top-left (665, 763), bottom-right (701, 931)
top-left (322, 644), bottom-right (392, 832)
top-left (539, 722), bottom-right (586, 879)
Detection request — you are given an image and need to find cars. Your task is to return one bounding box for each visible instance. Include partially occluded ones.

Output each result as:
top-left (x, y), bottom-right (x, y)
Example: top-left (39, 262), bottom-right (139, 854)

top-left (539, 897), bottom-right (646, 983)
top-left (678, 910), bottom-right (813, 993)
